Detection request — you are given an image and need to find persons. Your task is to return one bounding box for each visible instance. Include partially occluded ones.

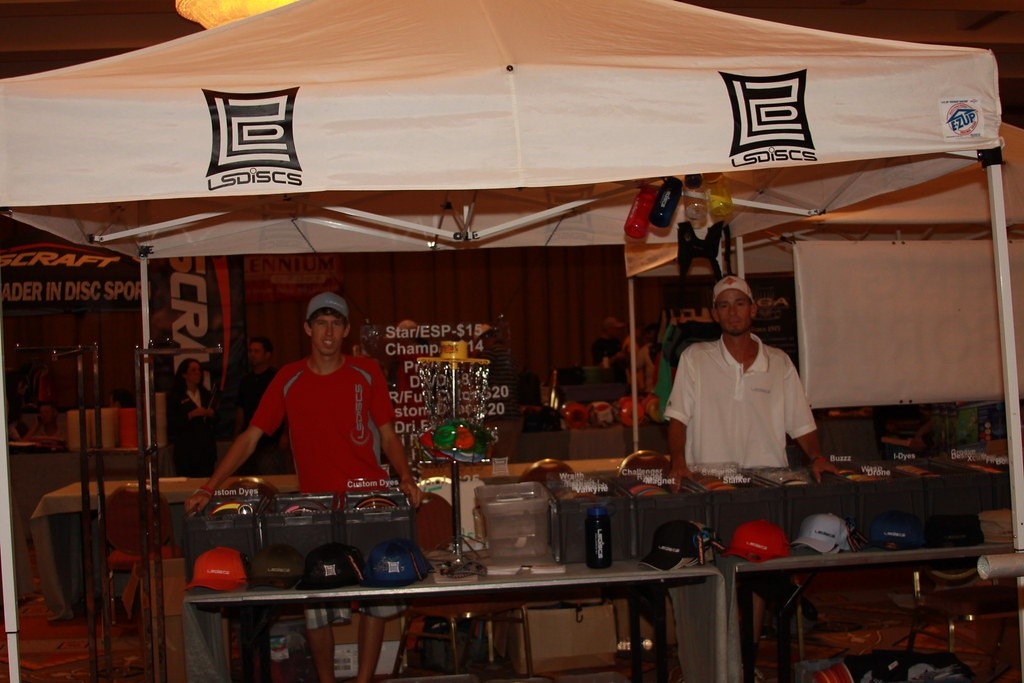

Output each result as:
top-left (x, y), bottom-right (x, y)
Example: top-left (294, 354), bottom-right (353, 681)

top-left (471, 324), bottom-right (522, 465)
top-left (183, 292), bottom-right (423, 683)
top-left (165, 358), bottom-right (218, 478)
top-left (232, 335), bottom-right (286, 476)
top-left (591, 316), bottom-right (662, 398)
top-left (385, 319), bottom-right (437, 437)
top-left (5, 371), bottom-right (132, 452)
top-left (663, 276), bottom-right (839, 683)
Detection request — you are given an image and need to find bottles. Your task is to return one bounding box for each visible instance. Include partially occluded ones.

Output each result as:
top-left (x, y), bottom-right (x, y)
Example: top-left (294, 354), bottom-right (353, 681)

top-left (585, 503), bottom-right (618, 569)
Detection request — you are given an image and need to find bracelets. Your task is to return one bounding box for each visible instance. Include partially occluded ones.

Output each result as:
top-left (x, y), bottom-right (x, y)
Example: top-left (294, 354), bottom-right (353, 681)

top-left (192, 486), bottom-right (215, 499)
top-left (812, 456), bottom-right (827, 464)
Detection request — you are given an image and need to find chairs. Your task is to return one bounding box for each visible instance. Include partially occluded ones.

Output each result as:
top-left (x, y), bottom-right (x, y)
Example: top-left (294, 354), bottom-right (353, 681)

top-left (387, 491), bottom-right (533, 683)
top-left (908, 565), bottom-right (1021, 683)
top-left (103, 477), bottom-right (174, 625)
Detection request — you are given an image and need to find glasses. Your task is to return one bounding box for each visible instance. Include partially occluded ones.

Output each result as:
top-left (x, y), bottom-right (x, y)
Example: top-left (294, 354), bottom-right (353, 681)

top-left (310, 319), bottom-right (346, 329)
top-left (717, 299), bottom-right (749, 311)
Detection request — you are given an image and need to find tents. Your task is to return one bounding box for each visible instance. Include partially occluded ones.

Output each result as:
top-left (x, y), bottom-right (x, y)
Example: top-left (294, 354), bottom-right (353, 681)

top-left (625, 121), bottom-right (1024, 450)
top-left (0, 0), bottom-right (1024, 683)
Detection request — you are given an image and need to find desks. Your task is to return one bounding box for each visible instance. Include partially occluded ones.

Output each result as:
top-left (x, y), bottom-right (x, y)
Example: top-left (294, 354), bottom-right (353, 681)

top-left (43, 474), bottom-right (300, 617)
top-left (715, 541), bottom-right (1013, 683)
top-left (183, 553), bottom-right (725, 683)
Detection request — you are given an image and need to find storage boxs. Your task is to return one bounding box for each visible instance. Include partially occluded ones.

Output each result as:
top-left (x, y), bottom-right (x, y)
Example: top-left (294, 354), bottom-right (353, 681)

top-left (179, 451), bottom-right (1008, 569)
top-left (334, 593), bottom-right (677, 679)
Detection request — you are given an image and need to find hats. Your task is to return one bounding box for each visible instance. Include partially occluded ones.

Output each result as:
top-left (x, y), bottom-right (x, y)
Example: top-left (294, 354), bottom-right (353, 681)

top-left (183, 546), bottom-right (250, 591)
top-left (720, 519), bottom-right (794, 563)
top-left (866, 510), bottom-right (926, 549)
top-left (474, 324), bottom-right (493, 341)
top-left (384, 320), bottom-right (422, 338)
top-left (923, 512), bottom-right (985, 549)
top-left (295, 544), bottom-right (367, 589)
top-left (360, 539), bottom-right (435, 587)
top-left (601, 316), bottom-right (625, 331)
top-left (306, 292), bottom-right (349, 322)
top-left (247, 544), bottom-right (304, 589)
top-left (790, 513), bottom-right (871, 555)
top-left (712, 275), bottom-right (754, 305)
top-left (639, 519), bottom-right (724, 570)
